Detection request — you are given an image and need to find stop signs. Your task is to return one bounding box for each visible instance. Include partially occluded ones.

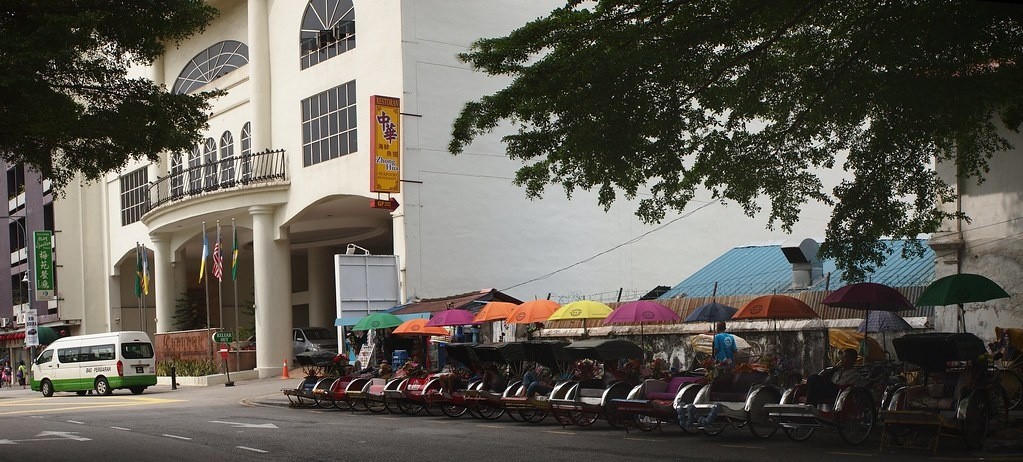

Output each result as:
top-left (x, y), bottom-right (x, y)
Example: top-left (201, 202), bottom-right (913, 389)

top-left (219, 343), bottom-right (229, 359)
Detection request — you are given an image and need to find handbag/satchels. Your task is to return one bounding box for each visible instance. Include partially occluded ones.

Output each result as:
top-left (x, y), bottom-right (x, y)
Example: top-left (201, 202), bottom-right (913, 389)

top-left (1, 369), bottom-right (6, 379)
top-left (17, 368), bottom-right (23, 378)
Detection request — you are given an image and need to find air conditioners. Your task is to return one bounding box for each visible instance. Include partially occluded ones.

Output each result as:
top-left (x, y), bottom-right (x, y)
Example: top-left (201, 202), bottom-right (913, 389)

top-left (1, 318), bottom-right (10, 328)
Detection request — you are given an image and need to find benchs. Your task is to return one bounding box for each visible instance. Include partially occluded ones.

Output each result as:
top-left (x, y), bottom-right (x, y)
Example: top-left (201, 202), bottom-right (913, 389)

top-left (303, 375), bottom-right (986, 421)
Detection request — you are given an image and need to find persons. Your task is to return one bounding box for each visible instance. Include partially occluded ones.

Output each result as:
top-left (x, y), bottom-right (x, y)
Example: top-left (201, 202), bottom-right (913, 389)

top-left (710, 321), bottom-right (738, 368)
top-left (0, 360), bottom-right (28, 389)
top-left (520, 357), bottom-right (554, 397)
top-left (481, 364), bottom-right (506, 394)
top-left (805, 348), bottom-right (857, 408)
top-left (438, 355), bottom-right (472, 393)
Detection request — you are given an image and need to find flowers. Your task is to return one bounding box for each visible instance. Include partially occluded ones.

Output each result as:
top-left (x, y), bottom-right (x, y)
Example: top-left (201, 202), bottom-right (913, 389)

top-left (977, 342), bottom-right (1001, 372)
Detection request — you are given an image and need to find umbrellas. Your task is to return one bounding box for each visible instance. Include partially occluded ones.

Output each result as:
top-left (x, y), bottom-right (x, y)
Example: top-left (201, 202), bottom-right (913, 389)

top-left (681, 298), bottom-right (738, 352)
top-left (819, 276), bottom-right (917, 364)
top-left (602, 297), bottom-right (681, 359)
top-left (351, 295), bottom-right (562, 341)
top-left (915, 274), bottom-right (1012, 333)
top-left (547, 295), bottom-right (613, 337)
top-left (731, 288), bottom-right (819, 345)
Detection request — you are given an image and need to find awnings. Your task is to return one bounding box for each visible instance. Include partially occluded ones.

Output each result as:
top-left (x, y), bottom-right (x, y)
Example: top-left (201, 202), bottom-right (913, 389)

top-left (335, 312), bottom-right (431, 326)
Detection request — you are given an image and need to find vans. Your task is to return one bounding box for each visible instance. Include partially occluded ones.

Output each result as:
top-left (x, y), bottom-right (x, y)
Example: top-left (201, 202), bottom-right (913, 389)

top-left (29, 330), bottom-right (158, 397)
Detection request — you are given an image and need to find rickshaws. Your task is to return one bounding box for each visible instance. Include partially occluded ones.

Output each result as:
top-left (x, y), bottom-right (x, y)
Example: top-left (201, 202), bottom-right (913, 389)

top-left (764, 338), bottom-right (905, 440)
top-left (878, 327), bottom-right (1023, 450)
top-left (285, 334), bottom-right (726, 434)
top-left (673, 356), bottom-right (815, 436)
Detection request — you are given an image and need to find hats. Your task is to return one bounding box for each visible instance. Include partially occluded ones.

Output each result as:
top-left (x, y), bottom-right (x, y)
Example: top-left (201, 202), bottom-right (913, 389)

top-left (718, 322), bottom-right (726, 329)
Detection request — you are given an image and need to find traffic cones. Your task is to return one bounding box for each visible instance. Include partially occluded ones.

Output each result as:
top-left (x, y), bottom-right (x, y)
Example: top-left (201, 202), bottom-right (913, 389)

top-left (281, 358), bottom-right (290, 379)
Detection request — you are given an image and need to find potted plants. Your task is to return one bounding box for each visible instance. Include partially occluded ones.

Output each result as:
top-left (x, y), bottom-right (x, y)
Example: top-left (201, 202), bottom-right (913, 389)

top-left (231, 327), bottom-right (255, 350)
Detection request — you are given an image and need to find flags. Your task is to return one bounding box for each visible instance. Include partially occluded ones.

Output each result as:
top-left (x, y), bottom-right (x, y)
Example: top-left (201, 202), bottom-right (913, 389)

top-left (199, 230), bottom-right (210, 284)
top-left (212, 229), bottom-right (224, 282)
top-left (134, 245), bottom-right (151, 299)
top-left (231, 224), bottom-right (239, 280)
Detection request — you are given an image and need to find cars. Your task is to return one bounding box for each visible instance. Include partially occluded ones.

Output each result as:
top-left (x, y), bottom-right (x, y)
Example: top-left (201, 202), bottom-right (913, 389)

top-left (242, 333), bottom-right (256, 350)
top-left (294, 328), bottom-right (338, 358)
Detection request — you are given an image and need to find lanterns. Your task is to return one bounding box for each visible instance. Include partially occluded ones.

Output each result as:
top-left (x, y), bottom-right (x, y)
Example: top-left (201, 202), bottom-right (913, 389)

top-left (0, 331), bottom-right (25, 344)
